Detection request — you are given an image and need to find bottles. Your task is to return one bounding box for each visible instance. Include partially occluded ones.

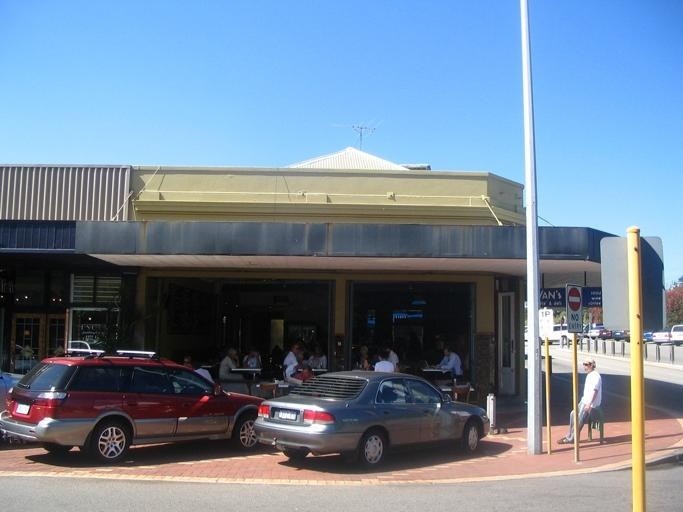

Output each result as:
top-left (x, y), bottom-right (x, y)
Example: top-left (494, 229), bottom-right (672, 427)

top-left (583, 360), bottom-right (596, 368)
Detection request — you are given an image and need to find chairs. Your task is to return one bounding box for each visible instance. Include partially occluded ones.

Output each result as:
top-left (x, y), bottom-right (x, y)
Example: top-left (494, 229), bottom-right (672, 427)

top-left (557, 437), bottom-right (570, 444)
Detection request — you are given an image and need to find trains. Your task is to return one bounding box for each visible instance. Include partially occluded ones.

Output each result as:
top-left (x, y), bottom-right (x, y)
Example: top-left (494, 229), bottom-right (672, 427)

top-left (565, 284), bottom-right (582, 333)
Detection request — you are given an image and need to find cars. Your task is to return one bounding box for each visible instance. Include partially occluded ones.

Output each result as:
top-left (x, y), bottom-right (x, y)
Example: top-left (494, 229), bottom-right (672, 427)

top-left (252, 370), bottom-right (490, 464)
top-left (553, 322), bottom-right (653, 347)
top-left (0, 347), bottom-right (269, 463)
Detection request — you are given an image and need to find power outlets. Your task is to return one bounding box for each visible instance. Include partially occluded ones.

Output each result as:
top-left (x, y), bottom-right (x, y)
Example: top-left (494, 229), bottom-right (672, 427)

top-left (654, 325), bottom-right (683, 345)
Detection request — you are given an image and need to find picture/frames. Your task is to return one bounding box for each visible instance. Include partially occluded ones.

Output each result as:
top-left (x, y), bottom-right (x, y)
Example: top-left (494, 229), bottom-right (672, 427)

top-left (231, 367), bottom-right (475, 404)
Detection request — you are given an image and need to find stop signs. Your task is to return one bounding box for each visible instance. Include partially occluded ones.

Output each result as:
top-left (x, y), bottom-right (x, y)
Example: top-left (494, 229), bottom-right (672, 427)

top-left (565, 284), bottom-right (582, 333)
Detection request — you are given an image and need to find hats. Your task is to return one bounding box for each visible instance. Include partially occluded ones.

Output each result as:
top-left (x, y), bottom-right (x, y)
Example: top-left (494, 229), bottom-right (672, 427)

top-left (553, 322), bottom-right (653, 347)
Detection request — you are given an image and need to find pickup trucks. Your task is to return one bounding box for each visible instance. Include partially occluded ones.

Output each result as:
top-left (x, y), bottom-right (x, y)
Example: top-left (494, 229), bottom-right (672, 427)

top-left (654, 325), bottom-right (683, 345)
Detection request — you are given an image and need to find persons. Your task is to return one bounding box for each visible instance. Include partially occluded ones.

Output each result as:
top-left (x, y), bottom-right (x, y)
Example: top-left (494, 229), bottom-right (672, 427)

top-left (558, 358), bottom-right (602, 444)
top-left (353, 343), bottom-right (407, 373)
top-left (182, 355), bottom-right (216, 384)
top-left (438, 345), bottom-right (463, 380)
top-left (218, 339), bottom-right (327, 391)
